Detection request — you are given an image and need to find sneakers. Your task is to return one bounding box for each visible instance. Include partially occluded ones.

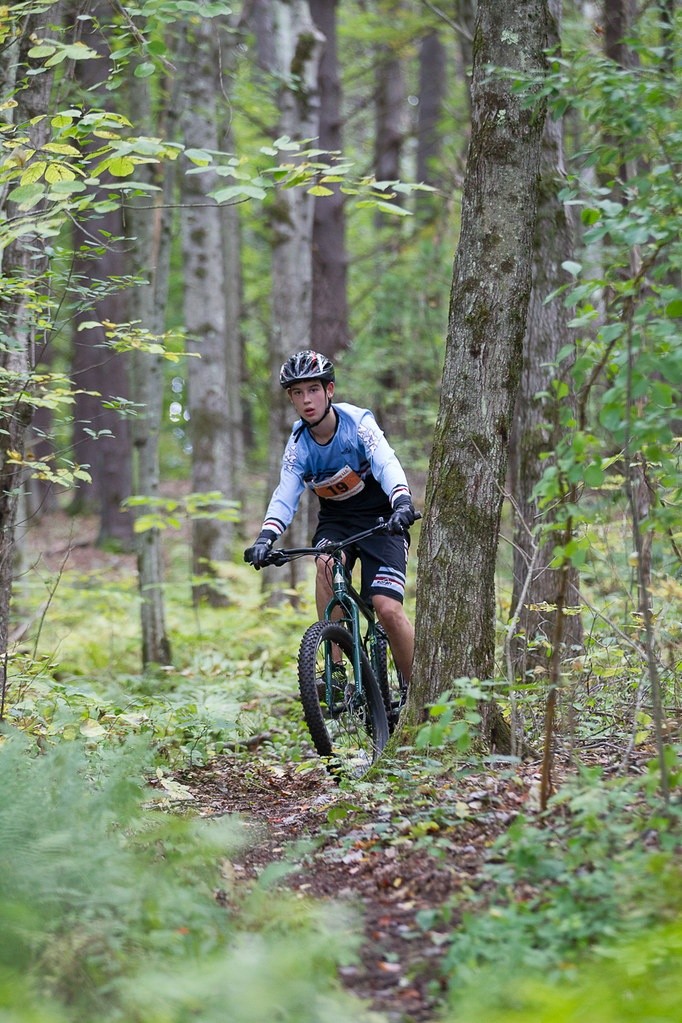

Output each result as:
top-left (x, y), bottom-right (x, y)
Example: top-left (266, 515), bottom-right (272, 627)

top-left (315, 660), bottom-right (349, 702)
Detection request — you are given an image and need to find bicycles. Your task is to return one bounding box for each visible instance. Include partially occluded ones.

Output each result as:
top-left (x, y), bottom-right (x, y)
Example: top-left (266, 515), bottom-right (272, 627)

top-left (248, 510), bottom-right (424, 783)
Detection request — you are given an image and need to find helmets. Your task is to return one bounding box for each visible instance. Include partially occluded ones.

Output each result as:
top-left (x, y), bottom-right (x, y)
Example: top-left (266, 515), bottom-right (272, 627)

top-left (279, 350), bottom-right (337, 388)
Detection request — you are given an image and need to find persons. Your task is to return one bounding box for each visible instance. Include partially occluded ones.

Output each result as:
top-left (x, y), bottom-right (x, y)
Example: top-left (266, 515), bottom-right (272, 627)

top-left (244, 350), bottom-right (415, 702)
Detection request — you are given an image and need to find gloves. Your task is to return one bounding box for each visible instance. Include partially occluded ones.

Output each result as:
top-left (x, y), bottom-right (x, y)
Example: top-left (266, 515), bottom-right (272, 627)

top-left (387, 504), bottom-right (415, 535)
top-left (243, 537), bottom-right (273, 570)
